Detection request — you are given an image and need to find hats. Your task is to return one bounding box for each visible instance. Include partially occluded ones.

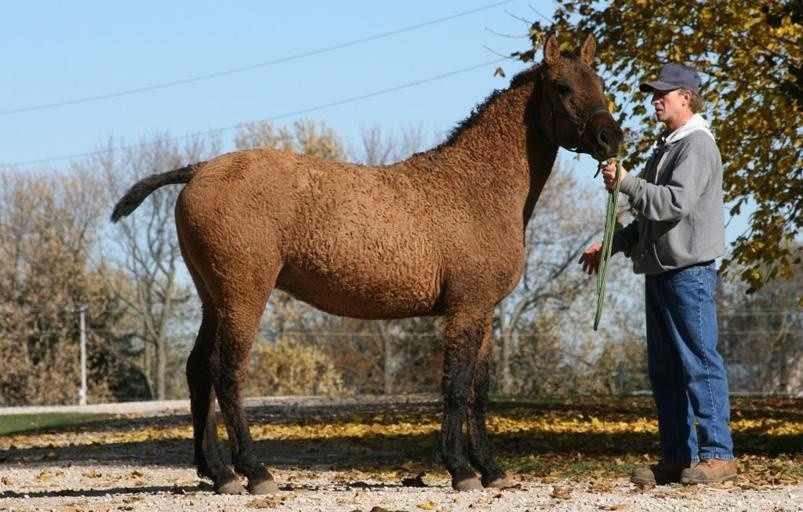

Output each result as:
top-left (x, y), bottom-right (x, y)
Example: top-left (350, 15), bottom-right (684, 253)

top-left (639, 62), bottom-right (702, 96)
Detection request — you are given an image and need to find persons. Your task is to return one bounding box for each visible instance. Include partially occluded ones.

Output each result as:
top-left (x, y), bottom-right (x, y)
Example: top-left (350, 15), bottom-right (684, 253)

top-left (577, 60), bottom-right (738, 485)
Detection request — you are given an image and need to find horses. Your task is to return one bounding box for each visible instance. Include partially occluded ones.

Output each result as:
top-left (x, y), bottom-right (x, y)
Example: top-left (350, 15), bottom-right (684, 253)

top-left (109, 29), bottom-right (626, 497)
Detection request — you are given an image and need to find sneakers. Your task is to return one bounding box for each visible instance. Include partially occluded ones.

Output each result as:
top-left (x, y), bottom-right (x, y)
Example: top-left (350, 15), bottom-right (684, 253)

top-left (682, 457), bottom-right (739, 486)
top-left (630, 456), bottom-right (690, 486)
